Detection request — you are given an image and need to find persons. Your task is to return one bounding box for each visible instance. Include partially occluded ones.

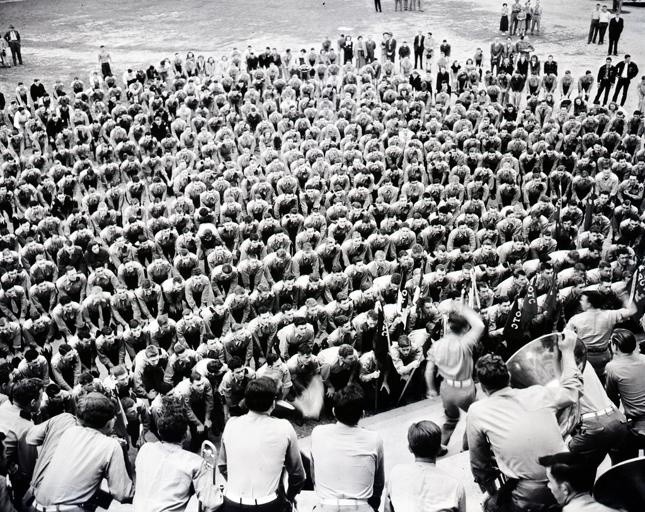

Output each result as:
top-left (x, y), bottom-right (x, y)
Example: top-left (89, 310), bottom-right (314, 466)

top-left (590, 2), bottom-right (624, 24)
top-left (567, 360), bottom-right (633, 473)
top-left (3, 25), bottom-right (645, 437)
top-left (372, 0), bottom-right (424, 12)
top-left (23, 412), bottom-right (81, 512)
top-left (421, 299), bottom-right (485, 451)
top-left (132, 409), bottom-right (223, 512)
top-left (309, 386), bottom-right (385, 512)
top-left (219, 376), bottom-right (307, 512)
top-left (0, 431), bottom-right (16, 512)
top-left (465, 329), bottom-right (584, 511)
top-left (31, 391), bottom-right (134, 512)
top-left (537, 453), bottom-right (623, 512)
top-left (1, 378), bottom-right (45, 476)
top-left (500, 0), bottom-right (542, 24)
top-left (384, 420), bottom-right (466, 512)
top-left (604, 328), bottom-right (645, 450)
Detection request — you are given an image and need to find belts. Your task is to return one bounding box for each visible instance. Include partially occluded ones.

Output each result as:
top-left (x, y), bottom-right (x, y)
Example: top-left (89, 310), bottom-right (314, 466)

top-left (320, 496), bottom-right (369, 505)
top-left (579, 403), bottom-right (619, 420)
top-left (585, 347), bottom-right (609, 353)
top-left (445, 378), bottom-right (474, 388)
top-left (31, 500), bottom-right (79, 512)
top-left (225, 491), bottom-right (278, 505)
